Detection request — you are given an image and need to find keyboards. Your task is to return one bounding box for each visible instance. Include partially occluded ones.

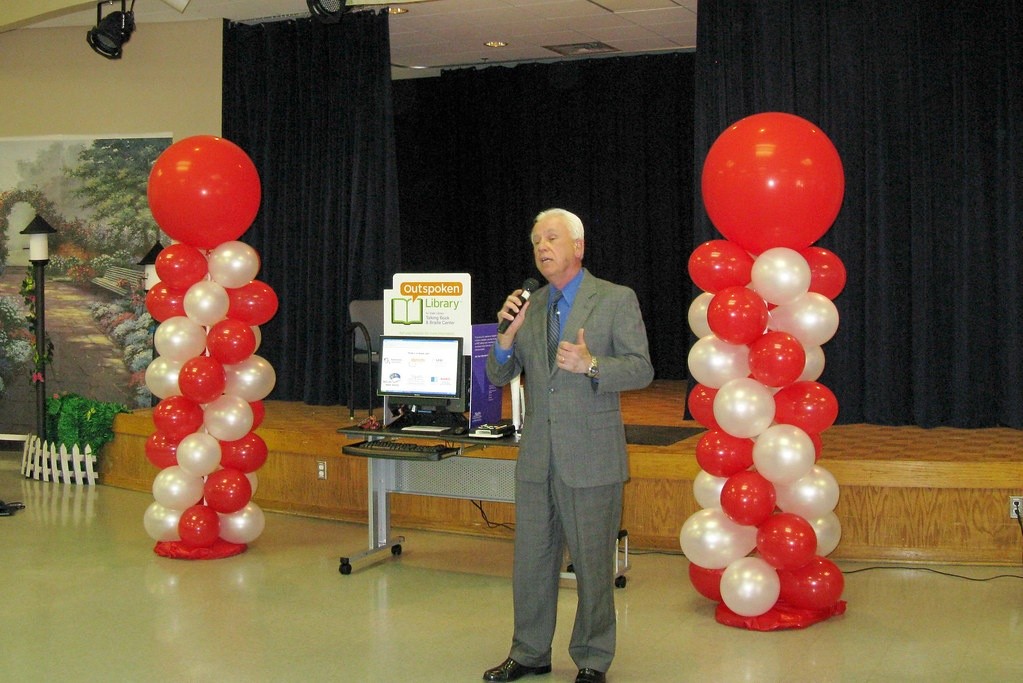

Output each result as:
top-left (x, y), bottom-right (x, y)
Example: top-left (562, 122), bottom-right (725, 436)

top-left (342, 440), bottom-right (459, 461)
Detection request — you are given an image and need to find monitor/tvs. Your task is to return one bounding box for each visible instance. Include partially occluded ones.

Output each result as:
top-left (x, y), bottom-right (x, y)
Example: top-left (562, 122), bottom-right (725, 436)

top-left (378, 336), bottom-right (463, 434)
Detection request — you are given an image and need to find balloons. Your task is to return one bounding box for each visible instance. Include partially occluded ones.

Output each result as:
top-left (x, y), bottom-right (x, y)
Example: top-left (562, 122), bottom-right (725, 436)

top-left (703, 113), bottom-right (846, 253)
top-left (679, 241), bottom-right (848, 614)
top-left (143, 240), bottom-right (278, 548)
top-left (147, 135), bottom-right (261, 250)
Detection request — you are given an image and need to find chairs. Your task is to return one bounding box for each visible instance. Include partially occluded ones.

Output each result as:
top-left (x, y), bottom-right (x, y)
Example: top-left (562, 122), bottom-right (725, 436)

top-left (347, 299), bottom-right (384, 420)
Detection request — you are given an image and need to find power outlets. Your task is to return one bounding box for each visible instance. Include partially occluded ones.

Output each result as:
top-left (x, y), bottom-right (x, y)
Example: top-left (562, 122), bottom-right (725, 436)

top-left (1009, 496), bottom-right (1023, 518)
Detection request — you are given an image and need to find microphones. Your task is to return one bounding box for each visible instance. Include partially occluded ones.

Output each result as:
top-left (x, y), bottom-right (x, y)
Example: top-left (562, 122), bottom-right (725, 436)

top-left (496, 277), bottom-right (539, 335)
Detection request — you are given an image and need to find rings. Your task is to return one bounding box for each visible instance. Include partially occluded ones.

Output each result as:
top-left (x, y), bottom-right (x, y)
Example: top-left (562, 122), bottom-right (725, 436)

top-left (561, 357), bottom-right (565, 363)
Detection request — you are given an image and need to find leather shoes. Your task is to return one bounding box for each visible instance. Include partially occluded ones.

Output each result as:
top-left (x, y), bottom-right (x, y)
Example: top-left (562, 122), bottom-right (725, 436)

top-left (483, 654), bottom-right (551, 682)
top-left (575, 667), bottom-right (605, 683)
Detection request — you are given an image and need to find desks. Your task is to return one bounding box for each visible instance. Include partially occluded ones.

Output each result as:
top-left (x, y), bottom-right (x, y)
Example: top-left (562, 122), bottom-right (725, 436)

top-left (335, 425), bottom-right (628, 590)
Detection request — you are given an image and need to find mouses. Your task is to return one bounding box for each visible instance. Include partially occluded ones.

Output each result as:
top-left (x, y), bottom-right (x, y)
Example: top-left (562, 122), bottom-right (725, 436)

top-left (454, 426), bottom-right (469, 435)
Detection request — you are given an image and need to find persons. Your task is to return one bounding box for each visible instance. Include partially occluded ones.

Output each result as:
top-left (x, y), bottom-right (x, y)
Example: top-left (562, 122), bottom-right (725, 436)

top-left (481, 209), bottom-right (655, 683)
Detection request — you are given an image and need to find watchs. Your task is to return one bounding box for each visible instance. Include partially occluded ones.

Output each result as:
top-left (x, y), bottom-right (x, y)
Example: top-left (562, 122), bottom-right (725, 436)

top-left (584, 356), bottom-right (599, 378)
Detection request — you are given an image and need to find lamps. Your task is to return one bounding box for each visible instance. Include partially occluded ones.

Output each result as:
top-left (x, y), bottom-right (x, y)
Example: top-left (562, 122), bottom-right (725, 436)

top-left (136, 240), bottom-right (167, 292)
top-left (20, 214), bottom-right (56, 265)
top-left (86, 0), bottom-right (137, 61)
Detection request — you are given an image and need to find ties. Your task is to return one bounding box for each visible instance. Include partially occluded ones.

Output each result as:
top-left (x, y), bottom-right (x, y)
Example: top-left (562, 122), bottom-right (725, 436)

top-left (547, 291), bottom-right (563, 372)
